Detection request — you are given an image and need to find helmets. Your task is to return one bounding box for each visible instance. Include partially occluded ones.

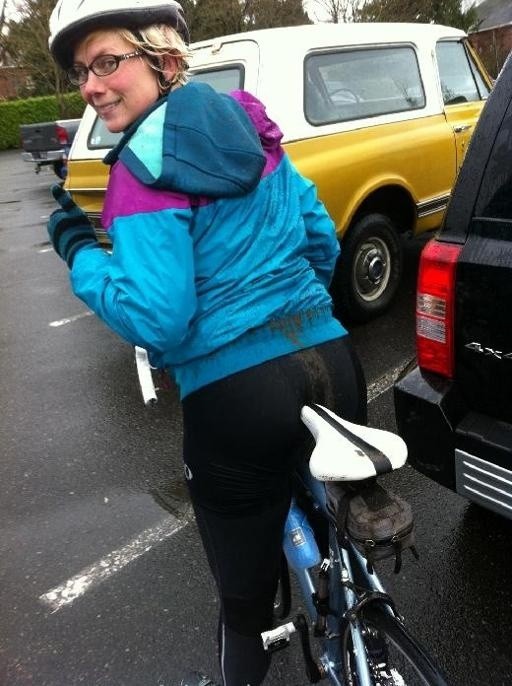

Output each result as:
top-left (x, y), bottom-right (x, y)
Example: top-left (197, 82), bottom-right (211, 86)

top-left (48, 0), bottom-right (191, 73)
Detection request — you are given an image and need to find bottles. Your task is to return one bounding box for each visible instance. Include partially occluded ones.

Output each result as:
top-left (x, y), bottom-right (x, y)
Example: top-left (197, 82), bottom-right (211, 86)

top-left (280, 497), bottom-right (322, 573)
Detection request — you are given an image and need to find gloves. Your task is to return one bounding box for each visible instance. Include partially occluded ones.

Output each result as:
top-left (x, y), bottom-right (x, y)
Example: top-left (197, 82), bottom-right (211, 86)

top-left (46, 183), bottom-right (98, 269)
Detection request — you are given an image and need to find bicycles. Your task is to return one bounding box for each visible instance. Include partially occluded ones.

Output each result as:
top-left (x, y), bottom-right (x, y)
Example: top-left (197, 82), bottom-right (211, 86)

top-left (125, 334), bottom-right (449, 686)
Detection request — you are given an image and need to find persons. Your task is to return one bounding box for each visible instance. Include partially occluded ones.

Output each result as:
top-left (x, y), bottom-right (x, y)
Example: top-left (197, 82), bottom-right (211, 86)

top-left (47, 0), bottom-right (368, 686)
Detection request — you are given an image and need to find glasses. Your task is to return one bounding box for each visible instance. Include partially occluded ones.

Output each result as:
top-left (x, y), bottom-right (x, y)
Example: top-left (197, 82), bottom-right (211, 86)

top-left (67, 51), bottom-right (143, 87)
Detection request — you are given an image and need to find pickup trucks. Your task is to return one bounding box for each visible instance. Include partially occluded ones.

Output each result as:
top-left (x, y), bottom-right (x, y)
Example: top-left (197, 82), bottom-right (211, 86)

top-left (15, 113), bottom-right (85, 182)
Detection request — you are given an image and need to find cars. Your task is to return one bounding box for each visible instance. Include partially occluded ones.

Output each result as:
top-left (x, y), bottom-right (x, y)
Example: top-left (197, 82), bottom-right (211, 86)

top-left (380, 42), bottom-right (512, 532)
top-left (54, 13), bottom-right (502, 330)
